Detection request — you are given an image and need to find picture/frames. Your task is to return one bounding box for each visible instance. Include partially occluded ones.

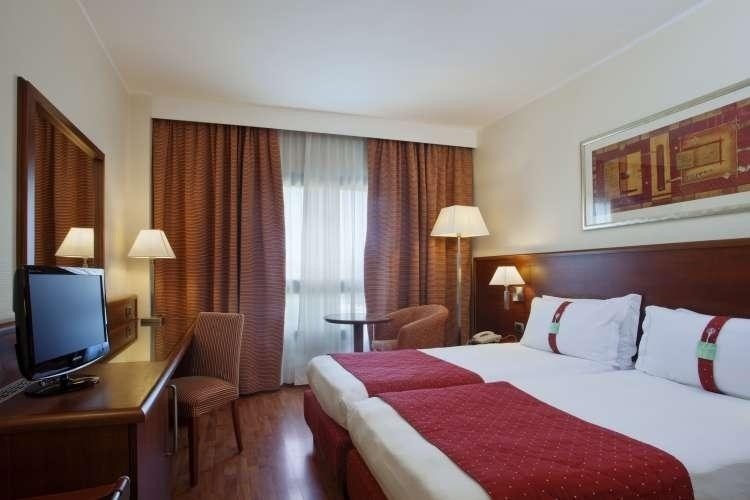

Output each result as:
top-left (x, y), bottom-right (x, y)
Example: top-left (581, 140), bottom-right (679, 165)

top-left (579, 77), bottom-right (750, 232)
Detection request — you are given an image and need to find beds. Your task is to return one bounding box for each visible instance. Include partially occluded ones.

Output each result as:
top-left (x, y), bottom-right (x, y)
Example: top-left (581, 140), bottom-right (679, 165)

top-left (304, 343), bottom-right (635, 472)
top-left (343, 369), bottom-right (748, 499)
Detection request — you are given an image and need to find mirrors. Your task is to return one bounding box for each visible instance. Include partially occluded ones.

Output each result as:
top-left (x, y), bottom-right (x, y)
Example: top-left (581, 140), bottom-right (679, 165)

top-left (18, 77), bottom-right (106, 272)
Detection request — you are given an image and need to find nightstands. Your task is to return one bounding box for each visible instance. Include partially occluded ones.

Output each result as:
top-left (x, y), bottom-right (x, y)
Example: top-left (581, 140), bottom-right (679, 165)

top-left (468, 334), bottom-right (519, 345)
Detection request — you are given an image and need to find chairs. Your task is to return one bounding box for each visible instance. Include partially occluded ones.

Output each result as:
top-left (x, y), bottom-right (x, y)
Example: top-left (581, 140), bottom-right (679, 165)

top-left (370, 304), bottom-right (449, 351)
top-left (166, 312), bottom-right (245, 488)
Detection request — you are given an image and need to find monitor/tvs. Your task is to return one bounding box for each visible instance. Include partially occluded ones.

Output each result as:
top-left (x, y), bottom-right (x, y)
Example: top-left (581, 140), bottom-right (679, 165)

top-left (12, 264), bottom-right (111, 398)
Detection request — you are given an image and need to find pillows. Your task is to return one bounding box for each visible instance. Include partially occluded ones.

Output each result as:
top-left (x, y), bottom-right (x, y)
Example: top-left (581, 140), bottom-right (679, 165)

top-left (634, 305), bottom-right (750, 400)
top-left (520, 294), bottom-right (643, 371)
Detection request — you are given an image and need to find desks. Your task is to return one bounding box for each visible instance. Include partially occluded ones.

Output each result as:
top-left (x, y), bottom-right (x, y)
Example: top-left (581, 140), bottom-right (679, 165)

top-left (323, 312), bottom-right (395, 352)
top-left (0, 293), bottom-right (199, 500)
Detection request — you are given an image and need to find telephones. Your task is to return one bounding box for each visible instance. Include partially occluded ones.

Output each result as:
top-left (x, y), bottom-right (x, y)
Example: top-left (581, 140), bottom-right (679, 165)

top-left (471, 331), bottom-right (502, 344)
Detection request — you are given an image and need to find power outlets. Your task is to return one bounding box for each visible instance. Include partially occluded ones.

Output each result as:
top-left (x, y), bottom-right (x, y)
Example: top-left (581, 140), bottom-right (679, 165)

top-left (514, 322), bottom-right (524, 334)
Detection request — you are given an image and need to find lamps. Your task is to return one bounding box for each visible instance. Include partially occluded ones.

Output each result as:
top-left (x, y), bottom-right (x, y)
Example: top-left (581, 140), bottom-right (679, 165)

top-left (127, 229), bottom-right (176, 324)
top-left (55, 226), bottom-right (94, 267)
top-left (488, 265), bottom-right (526, 310)
top-left (430, 204), bottom-right (491, 346)
